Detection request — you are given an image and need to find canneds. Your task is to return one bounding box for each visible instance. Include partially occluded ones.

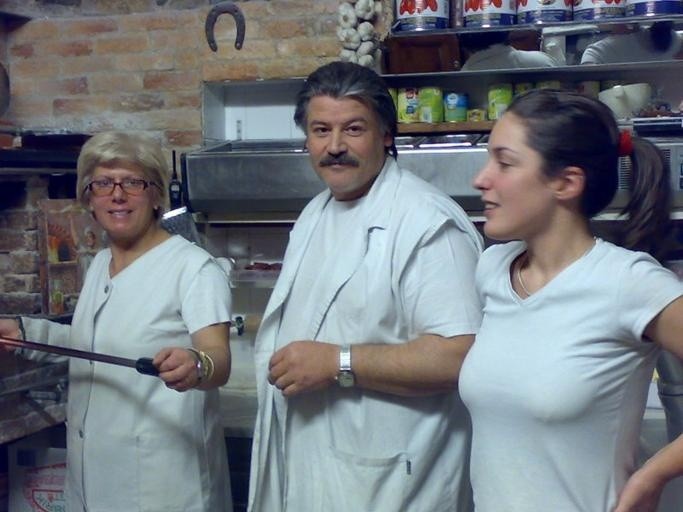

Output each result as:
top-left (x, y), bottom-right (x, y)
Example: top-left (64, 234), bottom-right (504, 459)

top-left (387, 76), bottom-right (626, 126)
top-left (396, 1), bottom-right (682, 32)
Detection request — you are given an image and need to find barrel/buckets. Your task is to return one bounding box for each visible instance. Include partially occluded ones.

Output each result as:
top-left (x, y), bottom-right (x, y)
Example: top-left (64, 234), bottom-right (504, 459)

top-left (393, 0), bottom-right (683, 33)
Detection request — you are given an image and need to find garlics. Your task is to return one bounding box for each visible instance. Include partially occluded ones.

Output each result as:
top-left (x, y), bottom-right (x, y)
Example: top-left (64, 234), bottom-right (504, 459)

top-left (335, 1), bottom-right (376, 68)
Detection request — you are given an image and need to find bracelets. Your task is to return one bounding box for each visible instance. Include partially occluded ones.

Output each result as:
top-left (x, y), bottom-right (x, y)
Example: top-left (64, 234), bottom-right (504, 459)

top-left (183, 347), bottom-right (216, 390)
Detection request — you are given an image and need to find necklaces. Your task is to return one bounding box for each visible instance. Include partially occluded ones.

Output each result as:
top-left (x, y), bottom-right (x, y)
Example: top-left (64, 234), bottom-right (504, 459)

top-left (516, 242), bottom-right (603, 297)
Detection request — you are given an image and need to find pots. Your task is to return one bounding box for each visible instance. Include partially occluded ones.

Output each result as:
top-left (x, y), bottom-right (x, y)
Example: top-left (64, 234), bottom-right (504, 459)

top-left (597, 82), bottom-right (652, 120)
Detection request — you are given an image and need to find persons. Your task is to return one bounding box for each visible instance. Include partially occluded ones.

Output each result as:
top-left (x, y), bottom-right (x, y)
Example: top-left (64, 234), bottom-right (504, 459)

top-left (239, 61), bottom-right (484, 512)
top-left (457, 32), bottom-right (566, 71)
top-left (580, 21), bottom-right (683, 107)
top-left (458, 89), bottom-right (683, 512)
top-left (1, 129), bottom-right (235, 512)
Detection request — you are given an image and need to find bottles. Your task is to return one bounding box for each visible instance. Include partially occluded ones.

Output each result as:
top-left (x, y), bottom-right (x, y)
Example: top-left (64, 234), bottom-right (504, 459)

top-left (168, 150), bottom-right (182, 212)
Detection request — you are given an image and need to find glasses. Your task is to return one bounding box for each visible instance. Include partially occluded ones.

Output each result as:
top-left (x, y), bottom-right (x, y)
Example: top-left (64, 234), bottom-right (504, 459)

top-left (81, 179), bottom-right (164, 199)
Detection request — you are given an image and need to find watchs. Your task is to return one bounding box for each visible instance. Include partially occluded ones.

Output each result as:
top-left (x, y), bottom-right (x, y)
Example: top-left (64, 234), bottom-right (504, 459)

top-left (332, 344), bottom-right (356, 393)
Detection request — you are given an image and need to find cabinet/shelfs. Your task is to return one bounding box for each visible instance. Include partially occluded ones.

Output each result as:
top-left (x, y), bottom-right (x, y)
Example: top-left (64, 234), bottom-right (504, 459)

top-left (379, 13), bottom-right (683, 134)
